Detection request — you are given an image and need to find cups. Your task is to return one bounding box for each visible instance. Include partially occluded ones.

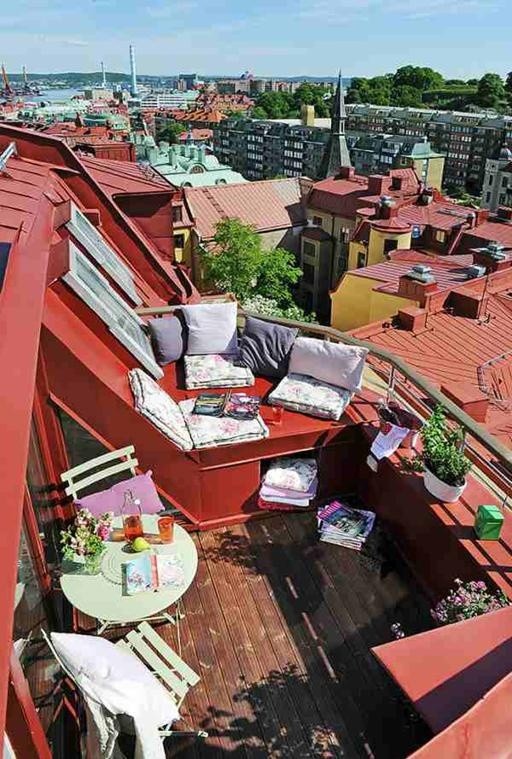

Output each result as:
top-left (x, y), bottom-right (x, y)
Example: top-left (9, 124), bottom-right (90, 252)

top-left (154, 512), bottom-right (175, 545)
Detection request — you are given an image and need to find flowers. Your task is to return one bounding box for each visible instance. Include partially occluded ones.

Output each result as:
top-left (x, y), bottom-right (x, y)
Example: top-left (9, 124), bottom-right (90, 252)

top-left (63, 509), bottom-right (113, 573)
top-left (390, 573), bottom-right (511, 640)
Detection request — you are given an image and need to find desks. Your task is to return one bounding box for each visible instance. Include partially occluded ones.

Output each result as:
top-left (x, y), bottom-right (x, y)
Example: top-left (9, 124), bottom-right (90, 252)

top-left (59, 514), bottom-right (199, 636)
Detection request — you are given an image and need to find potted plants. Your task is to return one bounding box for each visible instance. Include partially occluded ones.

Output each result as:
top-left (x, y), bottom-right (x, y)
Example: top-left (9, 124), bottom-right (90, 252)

top-left (399, 401), bottom-right (476, 503)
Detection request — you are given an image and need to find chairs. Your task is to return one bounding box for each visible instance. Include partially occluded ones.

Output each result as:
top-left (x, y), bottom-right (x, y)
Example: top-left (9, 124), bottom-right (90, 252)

top-left (41, 620), bottom-right (208, 759)
top-left (60, 444), bottom-right (165, 521)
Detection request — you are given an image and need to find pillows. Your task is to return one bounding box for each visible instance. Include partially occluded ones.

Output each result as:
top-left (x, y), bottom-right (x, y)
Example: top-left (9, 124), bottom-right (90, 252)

top-left (75, 469), bottom-right (166, 519)
top-left (48, 628), bottom-right (182, 726)
top-left (149, 306), bottom-right (370, 393)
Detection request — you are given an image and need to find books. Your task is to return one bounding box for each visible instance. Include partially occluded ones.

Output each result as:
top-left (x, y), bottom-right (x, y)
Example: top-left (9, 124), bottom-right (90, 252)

top-left (192, 390), bottom-right (261, 420)
top-left (126, 553), bottom-right (186, 595)
top-left (316, 501), bottom-right (376, 552)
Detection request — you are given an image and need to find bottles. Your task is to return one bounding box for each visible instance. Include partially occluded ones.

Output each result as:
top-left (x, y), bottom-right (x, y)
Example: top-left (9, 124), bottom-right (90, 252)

top-left (120, 488), bottom-right (145, 543)
top-left (377, 387), bottom-right (406, 428)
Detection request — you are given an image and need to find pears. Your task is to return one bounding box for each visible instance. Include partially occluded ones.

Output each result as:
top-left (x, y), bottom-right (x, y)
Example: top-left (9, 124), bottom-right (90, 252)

top-left (132, 536), bottom-right (152, 552)
